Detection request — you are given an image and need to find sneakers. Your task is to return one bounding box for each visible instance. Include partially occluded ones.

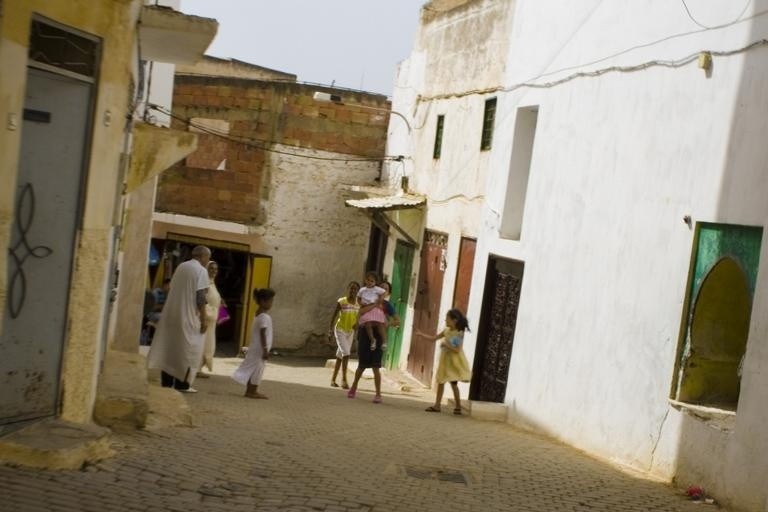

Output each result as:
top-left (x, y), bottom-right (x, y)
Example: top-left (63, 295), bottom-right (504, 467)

top-left (331, 383), bottom-right (356, 398)
top-left (245, 392), bottom-right (267, 398)
top-left (177, 386), bottom-right (197, 393)
top-left (196, 371), bottom-right (209, 378)
top-left (373, 396), bottom-right (381, 403)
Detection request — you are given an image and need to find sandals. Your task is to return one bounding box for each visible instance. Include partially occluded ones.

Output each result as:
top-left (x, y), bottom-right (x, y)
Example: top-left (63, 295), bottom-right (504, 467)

top-left (370, 338), bottom-right (386, 351)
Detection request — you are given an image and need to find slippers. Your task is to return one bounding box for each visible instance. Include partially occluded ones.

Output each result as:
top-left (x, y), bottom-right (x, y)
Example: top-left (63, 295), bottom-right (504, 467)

top-left (425, 407), bottom-right (440, 412)
top-left (454, 408), bottom-right (461, 414)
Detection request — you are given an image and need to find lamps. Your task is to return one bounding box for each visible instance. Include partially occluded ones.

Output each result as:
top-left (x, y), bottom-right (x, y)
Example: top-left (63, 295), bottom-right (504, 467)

top-left (314, 91), bottom-right (412, 132)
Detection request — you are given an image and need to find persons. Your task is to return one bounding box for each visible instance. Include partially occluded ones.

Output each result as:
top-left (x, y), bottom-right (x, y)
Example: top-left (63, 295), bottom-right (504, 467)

top-left (328, 281), bottom-right (360, 389)
top-left (355, 270), bottom-right (388, 352)
top-left (196, 260), bottom-right (228, 379)
top-left (414, 309), bottom-right (471, 415)
top-left (231, 287), bottom-right (275, 399)
top-left (347, 281), bottom-right (400, 403)
top-left (146, 278), bottom-right (171, 322)
top-left (147, 245), bottom-right (212, 393)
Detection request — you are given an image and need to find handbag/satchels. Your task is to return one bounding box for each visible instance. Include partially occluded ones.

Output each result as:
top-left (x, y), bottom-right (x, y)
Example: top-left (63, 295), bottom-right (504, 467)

top-left (217, 305), bottom-right (230, 326)
